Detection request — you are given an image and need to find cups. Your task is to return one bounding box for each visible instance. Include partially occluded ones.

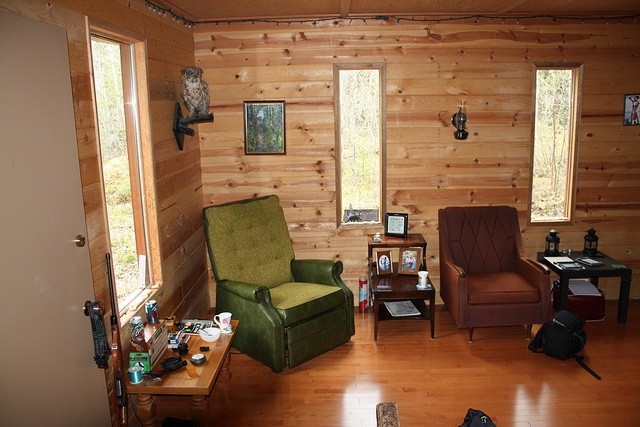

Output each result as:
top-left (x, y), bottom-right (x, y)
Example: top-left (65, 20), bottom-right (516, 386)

top-left (214, 312), bottom-right (232, 334)
top-left (418, 271), bottom-right (429, 287)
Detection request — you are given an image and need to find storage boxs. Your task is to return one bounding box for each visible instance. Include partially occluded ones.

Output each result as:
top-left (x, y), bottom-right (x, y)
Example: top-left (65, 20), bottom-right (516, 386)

top-left (551, 280), bottom-right (607, 323)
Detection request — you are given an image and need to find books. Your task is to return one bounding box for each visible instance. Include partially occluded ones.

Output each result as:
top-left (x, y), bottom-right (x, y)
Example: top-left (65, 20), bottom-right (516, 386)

top-left (384, 301), bottom-right (421, 316)
top-left (168, 330), bottom-right (190, 348)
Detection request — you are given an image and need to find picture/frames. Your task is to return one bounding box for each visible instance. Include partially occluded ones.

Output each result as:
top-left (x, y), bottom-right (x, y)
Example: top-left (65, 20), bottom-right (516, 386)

top-left (623, 93), bottom-right (640, 126)
top-left (384, 213), bottom-right (408, 238)
top-left (397, 247), bottom-right (421, 275)
top-left (376, 250), bottom-right (393, 276)
top-left (243, 100), bottom-right (286, 155)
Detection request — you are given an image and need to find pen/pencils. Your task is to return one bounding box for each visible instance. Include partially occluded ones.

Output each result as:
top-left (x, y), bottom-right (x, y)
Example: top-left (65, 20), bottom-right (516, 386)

top-left (552, 260), bottom-right (578, 265)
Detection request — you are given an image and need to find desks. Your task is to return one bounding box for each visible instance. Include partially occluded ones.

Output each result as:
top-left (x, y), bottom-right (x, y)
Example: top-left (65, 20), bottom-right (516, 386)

top-left (537, 251), bottom-right (632, 325)
top-left (125, 319), bottom-right (240, 424)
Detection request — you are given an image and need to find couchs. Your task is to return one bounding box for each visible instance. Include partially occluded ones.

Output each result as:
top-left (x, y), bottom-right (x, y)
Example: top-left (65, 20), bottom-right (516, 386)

top-left (438, 205), bottom-right (551, 344)
top-left (202, 194), bottom-right (355, 373)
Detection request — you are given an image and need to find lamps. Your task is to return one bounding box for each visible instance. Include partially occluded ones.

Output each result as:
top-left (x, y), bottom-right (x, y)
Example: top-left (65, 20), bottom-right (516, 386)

top-left (451, 99), bottom-right (469, 140)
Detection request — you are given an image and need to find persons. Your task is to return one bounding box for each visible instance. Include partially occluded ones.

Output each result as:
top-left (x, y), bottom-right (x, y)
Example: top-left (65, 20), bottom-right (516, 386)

top-left (380, 257), bottom-right (387, 270)
top-left (403, 254), bottom-right (416, 270)
top-left (628, 95), bottom-right (639, 125)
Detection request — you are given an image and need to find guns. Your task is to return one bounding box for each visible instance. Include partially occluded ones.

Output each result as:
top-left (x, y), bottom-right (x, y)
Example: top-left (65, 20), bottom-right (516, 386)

top-left (106, 253), bottom-right (132, 426)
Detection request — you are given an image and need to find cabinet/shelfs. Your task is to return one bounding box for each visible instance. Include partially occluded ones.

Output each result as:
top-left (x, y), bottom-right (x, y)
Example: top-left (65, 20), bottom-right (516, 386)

top-left (368, 233), bottom-right (436, 342)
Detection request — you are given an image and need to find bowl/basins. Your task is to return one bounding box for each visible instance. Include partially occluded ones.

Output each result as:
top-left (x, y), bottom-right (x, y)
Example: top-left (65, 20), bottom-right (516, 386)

top-left (199, 327), bottom-right (221, 342)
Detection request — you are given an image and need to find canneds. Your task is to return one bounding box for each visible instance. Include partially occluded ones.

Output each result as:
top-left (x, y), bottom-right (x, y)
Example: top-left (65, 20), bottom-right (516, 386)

top-left (145, 300), bottom-right (159, 324)
top-left (130, 316), bottom-right (145, 342)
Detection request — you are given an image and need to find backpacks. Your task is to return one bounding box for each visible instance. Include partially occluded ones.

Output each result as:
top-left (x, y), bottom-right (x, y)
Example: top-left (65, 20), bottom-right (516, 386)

top-left (528, 310), bottom-right (601, 380)
